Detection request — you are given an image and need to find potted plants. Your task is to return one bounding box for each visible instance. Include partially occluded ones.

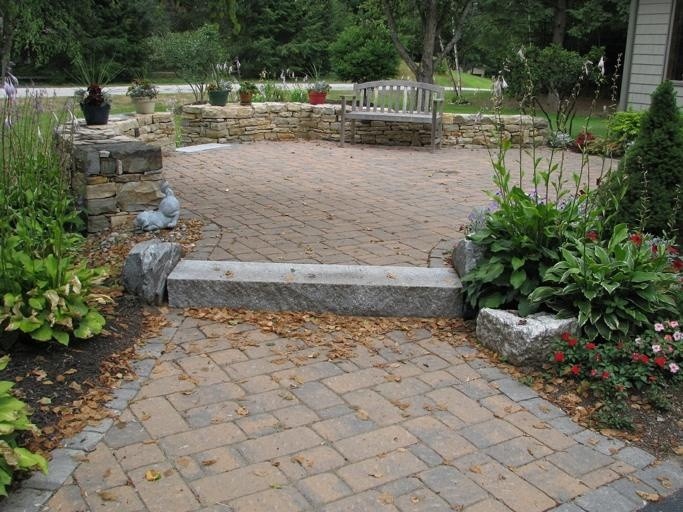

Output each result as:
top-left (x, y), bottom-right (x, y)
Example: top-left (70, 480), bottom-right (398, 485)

top-left (206, 80), bottom-right (233, 105)
top-left (125, 78), bottom-right (162, 114)
top-left (75, 84), bottom-right (112, 125)
top-left (236, 81), bottom-right (261, 105)
top-left (307, 81), bottom-right (332, 105)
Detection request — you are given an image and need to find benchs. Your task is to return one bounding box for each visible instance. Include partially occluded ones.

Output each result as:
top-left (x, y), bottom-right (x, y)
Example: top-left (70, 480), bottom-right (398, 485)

top-left (340, 79), bottom-right (445, 154)
